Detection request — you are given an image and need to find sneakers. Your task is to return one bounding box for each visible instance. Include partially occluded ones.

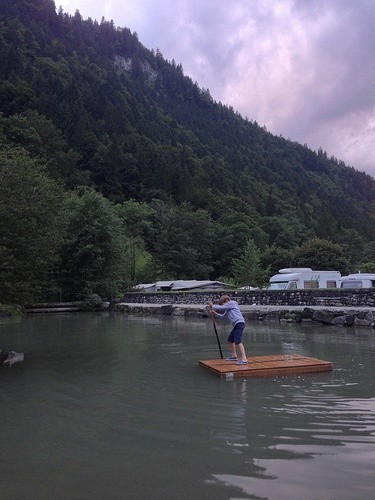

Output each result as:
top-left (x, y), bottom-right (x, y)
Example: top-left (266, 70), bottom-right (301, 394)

top-left (235, 359), bottom-right (248, 364)
top-left (225, 355), bottom-right (238, 361)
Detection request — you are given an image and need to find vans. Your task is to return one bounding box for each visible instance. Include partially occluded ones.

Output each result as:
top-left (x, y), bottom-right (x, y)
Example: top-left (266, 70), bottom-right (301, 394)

top-left (266, 267), bottom-right (341, 290)
top-left (339, 273), bottom-right (375, 290)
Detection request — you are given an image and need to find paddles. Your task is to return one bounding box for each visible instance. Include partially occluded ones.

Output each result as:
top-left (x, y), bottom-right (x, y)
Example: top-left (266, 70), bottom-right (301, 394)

top-left (206, 300), bottom-right (223, 360)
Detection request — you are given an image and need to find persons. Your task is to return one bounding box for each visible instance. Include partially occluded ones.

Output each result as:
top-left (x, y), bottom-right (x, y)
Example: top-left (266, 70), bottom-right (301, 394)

top-left (207, 295), bottom-right (248, 365)
